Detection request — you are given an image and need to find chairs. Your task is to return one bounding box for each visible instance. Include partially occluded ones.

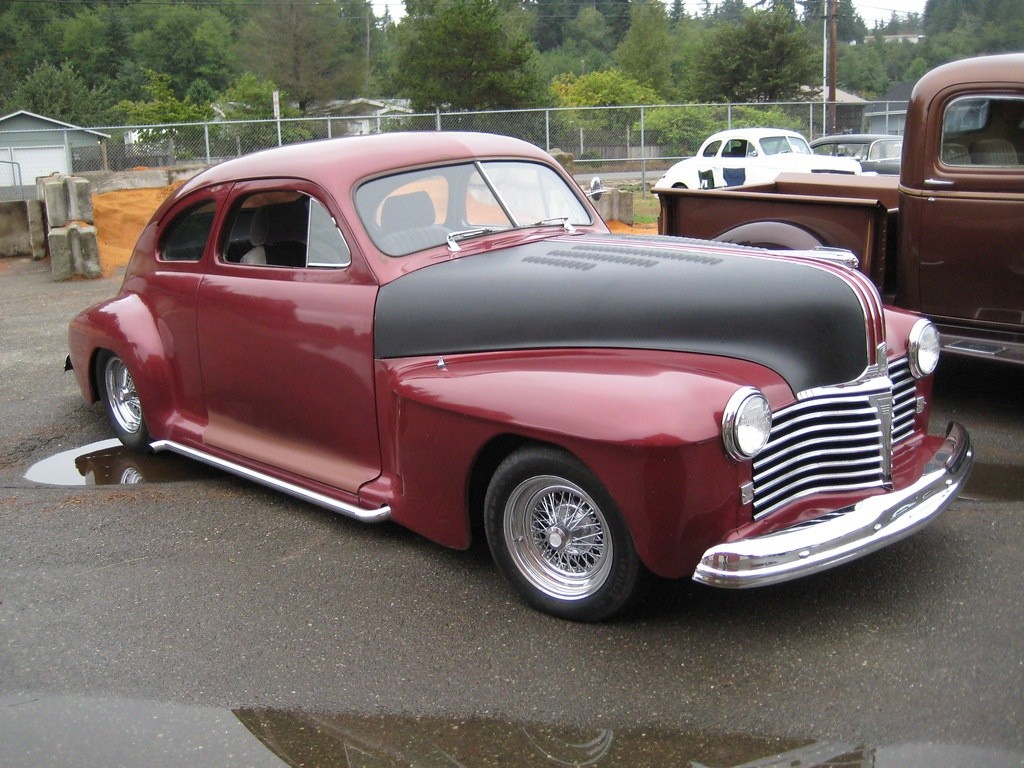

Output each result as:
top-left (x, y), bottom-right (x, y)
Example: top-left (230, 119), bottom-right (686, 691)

top-left (376, 191), bottom-right (457, 256)
top-left (943, 143), bottom-right (971, 165)
top-left (976, 139), bottom-right (1019, 165)
top-left (239, 204), bottom-right (332, 268)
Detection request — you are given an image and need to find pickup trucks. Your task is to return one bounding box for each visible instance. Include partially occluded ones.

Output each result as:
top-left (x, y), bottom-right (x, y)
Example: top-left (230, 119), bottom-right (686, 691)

top-left (651, 51), bottom-right (1024, 368)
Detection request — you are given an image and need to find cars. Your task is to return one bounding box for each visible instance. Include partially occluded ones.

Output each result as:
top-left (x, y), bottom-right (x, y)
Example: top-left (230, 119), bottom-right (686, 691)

top-left (63, 130), bottom-right (976, 623)
top-left (808, 135), bottom-right (905, 177)
top-left (649, 128), bottom-right (866, 214)
